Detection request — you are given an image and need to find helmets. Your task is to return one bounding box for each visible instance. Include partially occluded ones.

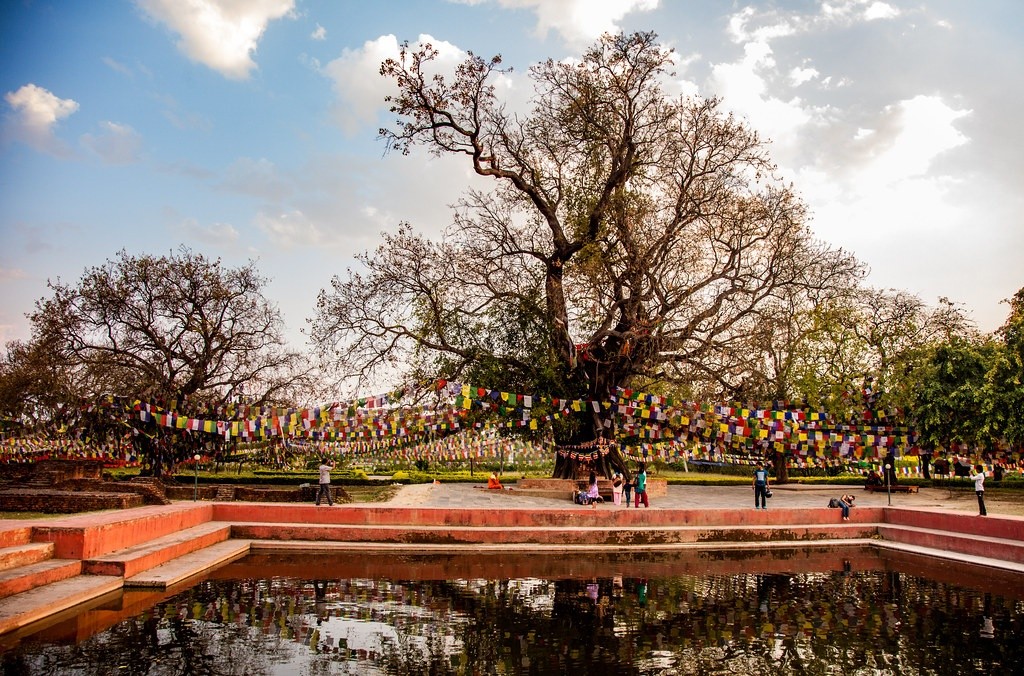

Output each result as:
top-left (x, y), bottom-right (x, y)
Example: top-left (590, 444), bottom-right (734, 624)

top-left (766, 491), bottom-right (773, 498)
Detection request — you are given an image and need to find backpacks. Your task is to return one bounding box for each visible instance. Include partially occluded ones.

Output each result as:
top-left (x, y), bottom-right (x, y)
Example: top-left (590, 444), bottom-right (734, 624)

top-left (827, 497), bottom-right (839, 508)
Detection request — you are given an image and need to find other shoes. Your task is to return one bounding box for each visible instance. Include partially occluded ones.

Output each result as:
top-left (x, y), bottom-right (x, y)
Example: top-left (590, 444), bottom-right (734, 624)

top-left (331, 503), bottom-right (336, 506)
top-left (626, 503), bottom-right (630, 508)
top-left (316, 504), bottom-right (321, 506)
top-left (763, 506), bottom-right (767, 509)
top-left (843, 517), bottom-right (850, 520)
top-left (756, 506), bottom-right (760, 509)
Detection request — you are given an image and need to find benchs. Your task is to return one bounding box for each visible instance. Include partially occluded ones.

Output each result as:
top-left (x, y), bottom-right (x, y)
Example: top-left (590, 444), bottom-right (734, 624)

top-left (869, 485), bottom-right (919, 494)
top-left (572, 480), bottom-right (614, 503)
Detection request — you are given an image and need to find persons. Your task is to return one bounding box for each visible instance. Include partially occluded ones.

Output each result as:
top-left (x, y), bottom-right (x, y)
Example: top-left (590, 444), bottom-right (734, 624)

top-left (313, 580), bottom-right (329, 628)
top-left (316, 458), bottom-right (336, 506)
top-left (611, 469), bottom-right (623, 506)
top-left (622, 464), bottom-right (649, 508)
top-left (752, 461), bottom-right (769, 509)
top-left (839, 494), bottom-right (855, 521)
top-left (585, 573), bottom-right (648, 608)
top-left (979, 610), bottom-right (994, 642)
top-left (488, 471), bottom-right (503, 489)
top-left (841, 559), bottom-right (854, 582)
top-left (867, 470), bottom-right (883, 486)
top-left (587, 472), bottom-right (598, 498)
top-left (969, 465), bottom-right (987, 517)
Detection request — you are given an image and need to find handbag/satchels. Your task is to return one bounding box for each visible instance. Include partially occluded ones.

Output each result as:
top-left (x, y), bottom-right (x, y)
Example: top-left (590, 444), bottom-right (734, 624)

top-left (612, 474), bottom-right (622, 488)
top-left (633, 474), bottom-right (638, 487)
